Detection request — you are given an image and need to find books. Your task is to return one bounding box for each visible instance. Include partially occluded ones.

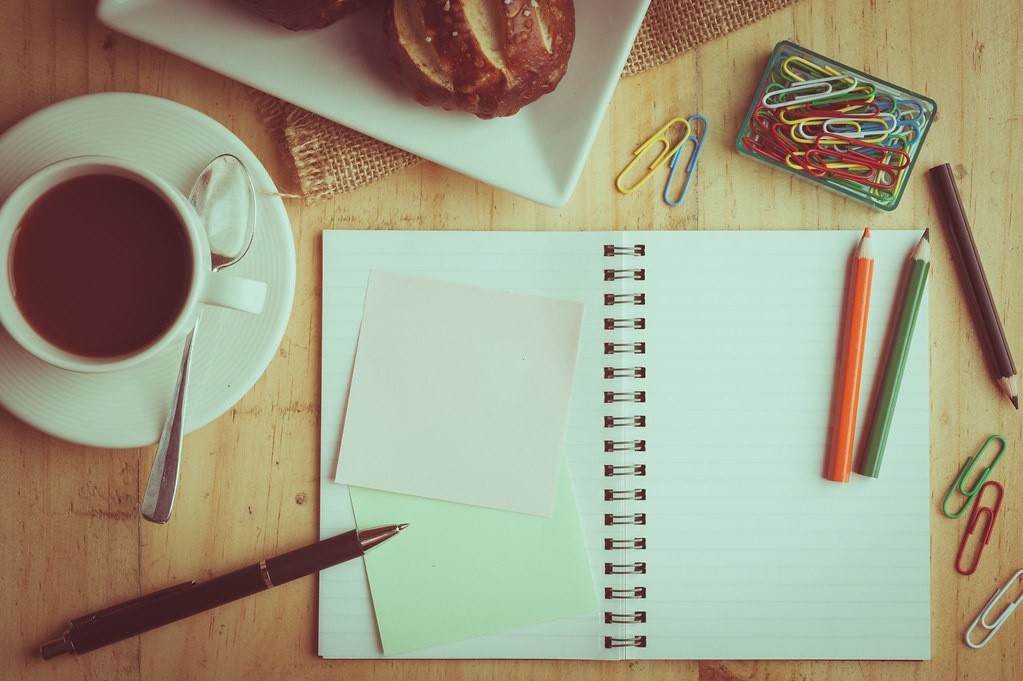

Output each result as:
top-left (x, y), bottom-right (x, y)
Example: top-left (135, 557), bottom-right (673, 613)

top-left (318, 229), bottom-right (932, 662)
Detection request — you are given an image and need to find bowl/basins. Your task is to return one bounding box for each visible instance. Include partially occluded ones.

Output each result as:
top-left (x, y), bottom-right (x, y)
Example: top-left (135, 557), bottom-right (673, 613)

top-left (1, 154), bottom-right (212, 375)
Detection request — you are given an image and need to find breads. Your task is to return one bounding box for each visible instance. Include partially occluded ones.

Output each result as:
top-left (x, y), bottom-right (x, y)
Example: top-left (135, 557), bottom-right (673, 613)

top-left (235, 0), bottom-right (378, 32)
top-left (380, 0), bottom-right (576, 120)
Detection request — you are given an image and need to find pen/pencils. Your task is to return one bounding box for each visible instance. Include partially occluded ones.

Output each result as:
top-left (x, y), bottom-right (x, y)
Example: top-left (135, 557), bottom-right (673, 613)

top-left (856, 228), bottom-right (933, 481)
top-left (35, 520), bottom-right (413, 659)
top-left (823, 228), bottom-right (874, 485)
top-left (927, 163), bottom-right (1021, 414)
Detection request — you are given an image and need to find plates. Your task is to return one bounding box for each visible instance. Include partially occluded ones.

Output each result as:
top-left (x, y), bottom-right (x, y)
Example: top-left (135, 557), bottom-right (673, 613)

top-left (97, 0), bottom-right (653, 210)
top-left (0, 90), bottom-right (296, 449)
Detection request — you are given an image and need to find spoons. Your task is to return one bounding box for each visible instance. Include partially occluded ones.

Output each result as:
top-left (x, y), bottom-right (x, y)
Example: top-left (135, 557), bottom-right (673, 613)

top-left (140, 154), bottom-right (257, 524)
